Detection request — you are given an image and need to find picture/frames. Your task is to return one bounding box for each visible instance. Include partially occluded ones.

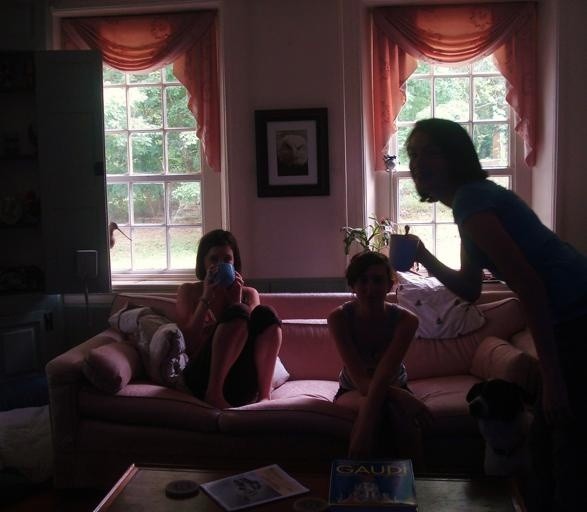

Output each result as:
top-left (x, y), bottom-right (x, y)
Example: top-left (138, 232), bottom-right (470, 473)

top-left (251, 105), bottom-right (330, 198)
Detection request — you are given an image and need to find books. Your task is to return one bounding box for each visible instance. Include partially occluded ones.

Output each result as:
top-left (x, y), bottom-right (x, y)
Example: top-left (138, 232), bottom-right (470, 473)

top-left (327, 458), bottom-right (419, 510)
top-left (199, 464), bottom-right (311, 511)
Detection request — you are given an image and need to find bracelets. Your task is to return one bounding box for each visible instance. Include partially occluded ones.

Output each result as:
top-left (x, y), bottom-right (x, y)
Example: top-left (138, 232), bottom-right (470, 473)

top-left (198, 295), bottom-right (210, 311)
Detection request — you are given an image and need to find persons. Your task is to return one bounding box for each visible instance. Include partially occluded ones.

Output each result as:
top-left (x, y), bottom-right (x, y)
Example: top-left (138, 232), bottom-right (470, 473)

top-left (325, 249), bottom-right (456, 475)
top-left (404, 116), bottom-right (587, 512)
top-left (173, 227), bottom-right (283, 412)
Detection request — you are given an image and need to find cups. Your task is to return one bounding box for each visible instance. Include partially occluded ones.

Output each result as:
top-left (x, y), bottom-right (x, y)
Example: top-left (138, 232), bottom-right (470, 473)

top-left (389, 234), bottom-right (420, 272)
top-left (212, 263), bottom-right (236, 287)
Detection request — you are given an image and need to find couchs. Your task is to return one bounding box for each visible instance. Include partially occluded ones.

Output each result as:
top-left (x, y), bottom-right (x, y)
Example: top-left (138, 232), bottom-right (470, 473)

top-left (43, 294), bottom-right (544, 493)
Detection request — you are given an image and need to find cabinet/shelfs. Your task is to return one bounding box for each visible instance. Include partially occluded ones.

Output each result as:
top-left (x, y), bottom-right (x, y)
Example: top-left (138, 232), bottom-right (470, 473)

top-left (0, 290), bottom-right (64, 409)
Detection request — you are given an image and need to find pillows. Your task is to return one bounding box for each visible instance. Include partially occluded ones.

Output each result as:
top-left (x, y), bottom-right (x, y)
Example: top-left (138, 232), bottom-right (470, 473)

top-left (82, 297), bottom-right (542, 402)
